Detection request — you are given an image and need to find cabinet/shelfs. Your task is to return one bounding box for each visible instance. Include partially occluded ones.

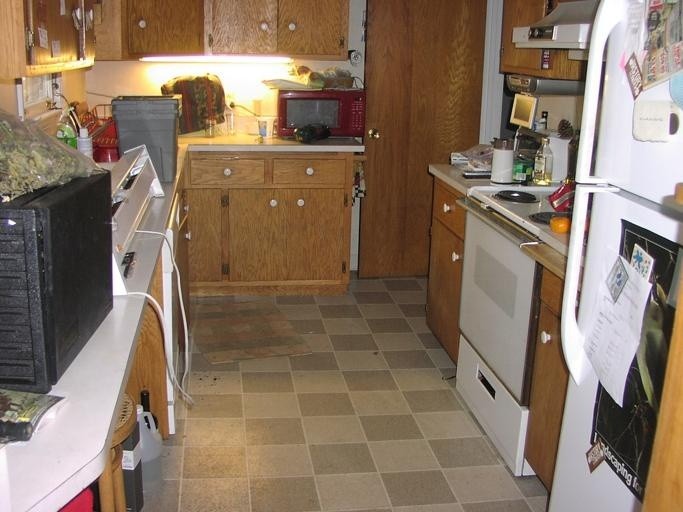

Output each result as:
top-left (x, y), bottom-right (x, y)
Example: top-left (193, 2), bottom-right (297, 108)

top-left (500, 3), bottom-right (586, 81)
top-left (0, 1), bottom-right (95, 79)
top-left (423, 175), bottom-right (465, 365)
top-left (98, 185), bottom-right (183, 511)
top-left (120, 1), bottom-right (209, 61)
top-left (524, 267), bottom-right (571, 493)
top-left (209, 1), bottom-right (349, 61)
top-left (186, 147), bottom-right (355, 298)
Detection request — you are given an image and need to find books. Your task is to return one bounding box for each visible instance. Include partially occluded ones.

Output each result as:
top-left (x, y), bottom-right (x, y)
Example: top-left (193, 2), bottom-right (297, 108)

top-left (0, 386), bottom-right (67, 455)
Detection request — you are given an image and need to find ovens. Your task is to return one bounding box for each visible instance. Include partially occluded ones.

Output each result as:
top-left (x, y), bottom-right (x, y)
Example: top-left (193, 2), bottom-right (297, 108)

top-left (1, 171), bottom-right (114, 395)
top-left (455, 211), bottom-right (540, 478)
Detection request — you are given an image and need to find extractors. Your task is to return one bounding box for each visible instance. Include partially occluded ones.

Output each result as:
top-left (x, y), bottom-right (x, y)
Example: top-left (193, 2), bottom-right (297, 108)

top-left (512, 1), bottom-right (601, 50)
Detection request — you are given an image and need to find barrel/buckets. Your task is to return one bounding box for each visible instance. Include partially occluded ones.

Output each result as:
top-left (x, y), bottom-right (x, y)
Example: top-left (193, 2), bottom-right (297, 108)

top-left (135, 404), bottom-right (165, 499)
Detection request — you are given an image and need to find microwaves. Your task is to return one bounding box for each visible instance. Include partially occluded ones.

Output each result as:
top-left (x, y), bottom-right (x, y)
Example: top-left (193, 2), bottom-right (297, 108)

top-left (276, 89), bottom-right (365, 139)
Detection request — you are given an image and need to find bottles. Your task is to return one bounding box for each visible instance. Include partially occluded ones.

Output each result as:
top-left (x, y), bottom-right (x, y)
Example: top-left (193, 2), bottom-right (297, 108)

top-left (532, 137), bottom-right (553, 187)
top-left (135, 391), bottom-right (159, 437)
top-left (491, 137), bottom-right (514, 184)
top-left (76, 128), bottom-right (93, 163)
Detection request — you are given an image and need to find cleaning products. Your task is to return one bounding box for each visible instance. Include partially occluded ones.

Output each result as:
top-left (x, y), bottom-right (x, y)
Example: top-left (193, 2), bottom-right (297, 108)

top-left (53, 105), bottom-right (77, 149)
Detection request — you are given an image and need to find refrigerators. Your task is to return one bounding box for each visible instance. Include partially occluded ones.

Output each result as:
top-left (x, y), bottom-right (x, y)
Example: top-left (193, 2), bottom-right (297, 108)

top-left (548, 0), bottom-right (683, 512)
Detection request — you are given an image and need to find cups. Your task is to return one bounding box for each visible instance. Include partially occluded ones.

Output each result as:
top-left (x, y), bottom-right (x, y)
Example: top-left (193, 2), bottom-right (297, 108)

top-left (224, 111), bottom-right (238, 136)
top-left (205, 119), bottom-right (217, 138)
top-left (258, 120), bottom-right (267, 137)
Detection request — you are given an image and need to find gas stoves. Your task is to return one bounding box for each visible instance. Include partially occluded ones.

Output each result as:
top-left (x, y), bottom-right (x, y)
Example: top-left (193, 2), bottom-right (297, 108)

top-left (470, 185), bottom-right (576, 257)
